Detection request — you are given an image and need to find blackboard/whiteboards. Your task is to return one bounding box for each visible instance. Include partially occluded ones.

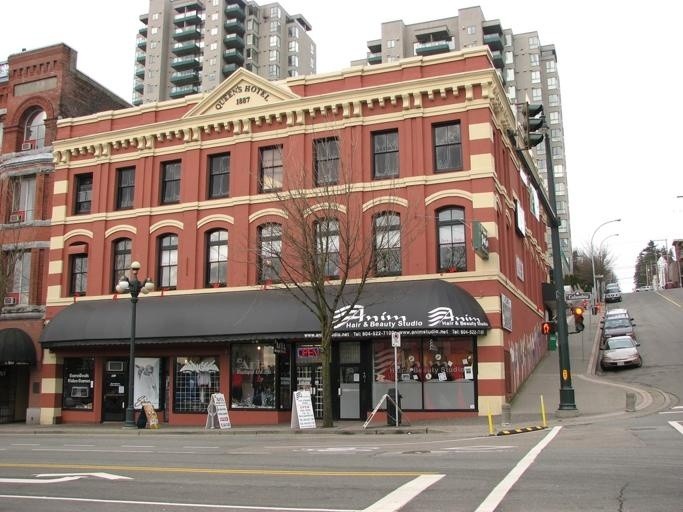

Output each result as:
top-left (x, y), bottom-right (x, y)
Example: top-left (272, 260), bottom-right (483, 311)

top-left (293, 392), bottom-right (317, 427)
top-left (210, 394), bottom-right (232, 427)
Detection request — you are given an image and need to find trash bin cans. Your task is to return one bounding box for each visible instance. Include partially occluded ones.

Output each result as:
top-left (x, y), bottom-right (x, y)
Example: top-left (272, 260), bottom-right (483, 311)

top-left (592, 307), bottom-right (597, 315)
top-left (548, 333), bottom-right (557, 351)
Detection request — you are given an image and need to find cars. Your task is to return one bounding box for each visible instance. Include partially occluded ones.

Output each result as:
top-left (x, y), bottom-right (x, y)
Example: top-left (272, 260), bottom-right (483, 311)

top-left (636, 286), bottom-right (654, 292)
top-left (600, 308), bottom-right (643, 370)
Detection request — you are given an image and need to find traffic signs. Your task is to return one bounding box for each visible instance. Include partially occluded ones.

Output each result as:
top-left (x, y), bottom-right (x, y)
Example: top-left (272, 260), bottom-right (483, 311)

top-left (565, 292), bottom-right (591, 300)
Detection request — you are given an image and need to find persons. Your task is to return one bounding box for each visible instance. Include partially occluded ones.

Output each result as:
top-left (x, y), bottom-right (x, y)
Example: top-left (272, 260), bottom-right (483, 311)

top-left (252, 367), bottom-right (264, 405)
top-left (231, 364), bottom-right (243, 402)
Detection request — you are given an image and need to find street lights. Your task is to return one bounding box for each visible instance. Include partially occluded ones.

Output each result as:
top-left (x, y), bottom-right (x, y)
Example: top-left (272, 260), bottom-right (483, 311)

top-left (643, 238), bottom-right (669, 290)
top-left (115, 261), bottom-right (155, 427)
top-left (591, 219), bottom-right (621, 307)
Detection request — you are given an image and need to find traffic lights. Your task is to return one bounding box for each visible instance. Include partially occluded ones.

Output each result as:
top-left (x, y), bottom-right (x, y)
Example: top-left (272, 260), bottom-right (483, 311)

top-left (572, 306), bottom-right (585, 331)
top-left (541, 321), bottom-right (555, 335)
top-left (522, 100), bottom-right (544, 149)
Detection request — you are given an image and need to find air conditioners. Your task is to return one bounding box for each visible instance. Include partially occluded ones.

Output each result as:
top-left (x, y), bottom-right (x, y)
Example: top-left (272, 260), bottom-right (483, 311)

top-left (9, 215), bottom-right (19, 223)
top-left (21, 142), bottom-right (31, 151)
top-left (4, 296), bottom-right (17, 306)
